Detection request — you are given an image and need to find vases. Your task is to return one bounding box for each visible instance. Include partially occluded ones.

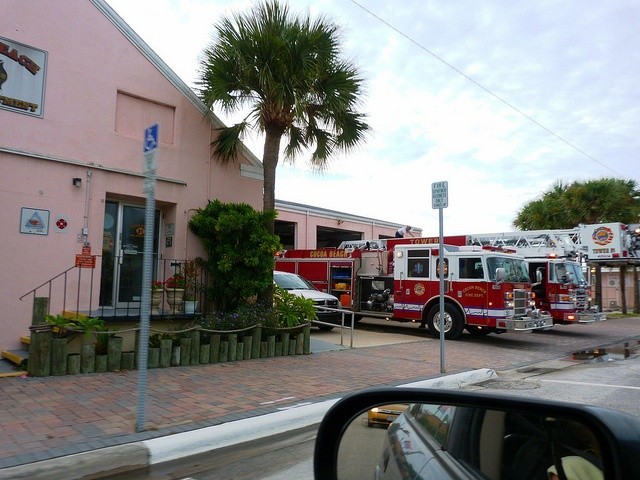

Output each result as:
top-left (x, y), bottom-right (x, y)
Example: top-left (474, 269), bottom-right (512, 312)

top-left (165, 289), bottom-right (184, 315)
top-left (152, 288), bottom-right (163, 315)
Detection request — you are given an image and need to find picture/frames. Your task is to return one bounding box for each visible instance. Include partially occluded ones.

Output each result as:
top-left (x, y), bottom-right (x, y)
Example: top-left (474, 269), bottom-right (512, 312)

top-left (19, 207), bottom-right (50, 235)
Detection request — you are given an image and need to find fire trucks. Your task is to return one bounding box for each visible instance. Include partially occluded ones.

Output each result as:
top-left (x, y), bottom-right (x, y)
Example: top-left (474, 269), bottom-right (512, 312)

top-left (338, 230), bottom-right (593, 325)
top-left (275, 243), bottom-right (555, 341)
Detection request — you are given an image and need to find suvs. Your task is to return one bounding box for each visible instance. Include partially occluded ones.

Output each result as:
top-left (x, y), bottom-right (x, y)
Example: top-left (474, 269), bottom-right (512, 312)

top-left (274, 271), bottom-right (341, 332)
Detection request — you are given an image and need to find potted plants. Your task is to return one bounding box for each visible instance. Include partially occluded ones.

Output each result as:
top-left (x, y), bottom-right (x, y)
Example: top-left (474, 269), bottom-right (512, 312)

top-left (94, 324), bottom-right (119, 355)
top-left (174, 258), bottom-right (205, 315)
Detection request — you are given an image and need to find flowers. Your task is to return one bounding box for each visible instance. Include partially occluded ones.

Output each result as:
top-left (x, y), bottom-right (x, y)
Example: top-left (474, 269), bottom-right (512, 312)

top-left (163, 273), bottom-right (188, 288)
top-left (153, 280), bottom-right (163, 288)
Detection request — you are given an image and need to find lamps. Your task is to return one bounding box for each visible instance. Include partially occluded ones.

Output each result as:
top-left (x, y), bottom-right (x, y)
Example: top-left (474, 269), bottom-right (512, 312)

top-left (73, 178), bottom-right (81, 187)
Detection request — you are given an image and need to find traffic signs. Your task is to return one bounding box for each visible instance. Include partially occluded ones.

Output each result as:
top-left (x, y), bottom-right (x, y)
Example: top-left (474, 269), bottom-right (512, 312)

top-left (432, 182), bottom-right (448, 209)
top-left (142, 124), bottom-right (158, 172)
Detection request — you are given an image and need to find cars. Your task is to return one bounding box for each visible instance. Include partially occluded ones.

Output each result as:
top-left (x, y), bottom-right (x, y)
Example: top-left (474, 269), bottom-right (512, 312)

top-left (367, 405), bottom-right (408, 427)
top-left (373, 404), bottom-right (605, 480)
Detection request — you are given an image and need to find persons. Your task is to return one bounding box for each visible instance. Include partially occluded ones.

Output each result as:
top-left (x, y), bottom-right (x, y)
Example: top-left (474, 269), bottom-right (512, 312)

top-left (395, 226), bottom-right (415, 239)
